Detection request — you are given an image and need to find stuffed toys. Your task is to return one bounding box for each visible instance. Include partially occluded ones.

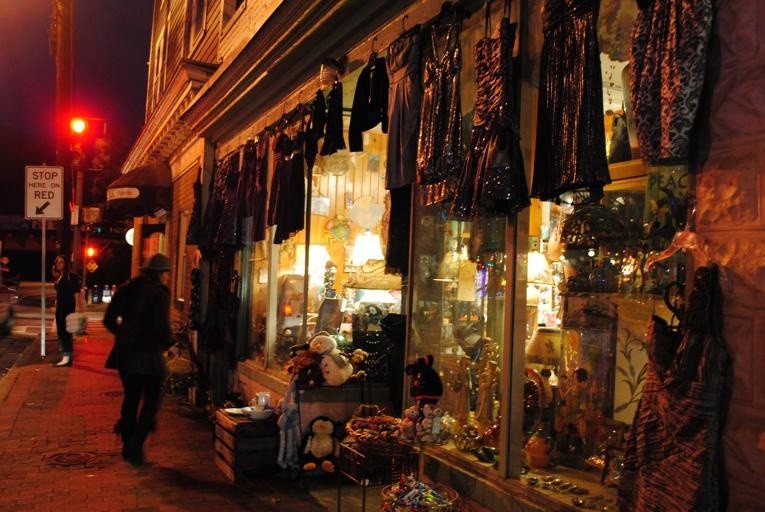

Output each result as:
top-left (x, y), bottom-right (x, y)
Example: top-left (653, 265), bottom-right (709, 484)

top-left (398, 353), bottom-right (444, 442)
top-left (271, 329), bottom-right (367, 477)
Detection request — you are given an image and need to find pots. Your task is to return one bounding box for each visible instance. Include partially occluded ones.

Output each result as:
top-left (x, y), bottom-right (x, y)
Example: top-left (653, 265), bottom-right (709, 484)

top-left (241, 406), bottom-right (273, 420)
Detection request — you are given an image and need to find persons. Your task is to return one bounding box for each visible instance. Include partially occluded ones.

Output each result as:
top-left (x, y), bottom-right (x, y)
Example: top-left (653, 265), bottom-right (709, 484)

top-left (102, 251), bottom-right (174, 466)
top-left (51, 254), bottom-right (81, 367)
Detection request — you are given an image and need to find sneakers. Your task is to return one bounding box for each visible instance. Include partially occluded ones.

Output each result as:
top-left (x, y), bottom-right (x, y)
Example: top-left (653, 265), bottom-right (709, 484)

top-left (116, 417), bottom-right (155, 465)
top-left (55, 355), bottom-right (71, 366)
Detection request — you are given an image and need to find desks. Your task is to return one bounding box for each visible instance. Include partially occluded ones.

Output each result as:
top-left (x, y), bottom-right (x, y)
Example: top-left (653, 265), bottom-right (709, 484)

top-left (335, 441), bottom-right (420, 512)
top-left (211, 406), bottom-right (282, 491)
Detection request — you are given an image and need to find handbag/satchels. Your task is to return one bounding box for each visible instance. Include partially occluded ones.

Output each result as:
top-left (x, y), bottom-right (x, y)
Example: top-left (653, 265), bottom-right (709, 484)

top-left (66, 311), bottom-right (88, 335)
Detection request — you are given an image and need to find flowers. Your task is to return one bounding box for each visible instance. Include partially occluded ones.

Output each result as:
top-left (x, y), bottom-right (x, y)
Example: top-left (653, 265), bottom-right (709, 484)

top-left (351, 402), bottom-right (403, 445)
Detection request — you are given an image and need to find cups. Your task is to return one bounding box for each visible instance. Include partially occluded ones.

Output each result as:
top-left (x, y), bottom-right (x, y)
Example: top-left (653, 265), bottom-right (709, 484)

top-left (254, 392), bottom-right (270, 409)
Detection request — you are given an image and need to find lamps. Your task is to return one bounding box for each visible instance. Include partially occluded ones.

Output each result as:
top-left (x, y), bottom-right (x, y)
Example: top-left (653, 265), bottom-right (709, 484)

top-left (525, 327), bottom-right (562, 409)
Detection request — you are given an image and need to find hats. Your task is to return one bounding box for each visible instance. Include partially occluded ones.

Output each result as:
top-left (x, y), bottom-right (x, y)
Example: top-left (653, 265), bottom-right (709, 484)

top-left (147, 253), bottom-right (171, 272)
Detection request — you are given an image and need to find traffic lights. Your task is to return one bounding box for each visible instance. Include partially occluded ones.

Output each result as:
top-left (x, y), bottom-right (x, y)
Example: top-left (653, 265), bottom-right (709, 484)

top-left (87, 246), bottom-right (95, 258)
top-left (69, 117), bottom-right (86, 154)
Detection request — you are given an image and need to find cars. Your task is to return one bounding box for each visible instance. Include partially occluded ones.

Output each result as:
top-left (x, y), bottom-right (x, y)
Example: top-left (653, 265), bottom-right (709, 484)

top-left (0, 283), bottom-right (17, 339)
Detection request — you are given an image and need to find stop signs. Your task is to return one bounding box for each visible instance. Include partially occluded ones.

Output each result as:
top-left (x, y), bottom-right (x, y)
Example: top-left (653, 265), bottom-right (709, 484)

top-left (23, 164), bottom-right (63, 221)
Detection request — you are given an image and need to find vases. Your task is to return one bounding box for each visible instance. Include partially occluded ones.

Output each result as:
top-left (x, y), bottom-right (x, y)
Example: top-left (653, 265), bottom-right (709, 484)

top-left (345, 416), bottom-right (402, 446)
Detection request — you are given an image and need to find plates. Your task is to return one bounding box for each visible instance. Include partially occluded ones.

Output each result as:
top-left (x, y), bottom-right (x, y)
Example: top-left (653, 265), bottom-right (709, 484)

top-left (224, 408), bottom-right (245, 415)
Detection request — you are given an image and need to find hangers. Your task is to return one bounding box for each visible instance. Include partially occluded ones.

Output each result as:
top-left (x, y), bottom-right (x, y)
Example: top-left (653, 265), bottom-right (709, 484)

top-left (645, 194), bottom-right (736, 294)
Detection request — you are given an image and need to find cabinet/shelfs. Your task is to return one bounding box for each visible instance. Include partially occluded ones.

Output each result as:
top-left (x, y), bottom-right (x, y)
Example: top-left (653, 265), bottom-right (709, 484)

top-left (560, 240), bottom-right (677, 300)
